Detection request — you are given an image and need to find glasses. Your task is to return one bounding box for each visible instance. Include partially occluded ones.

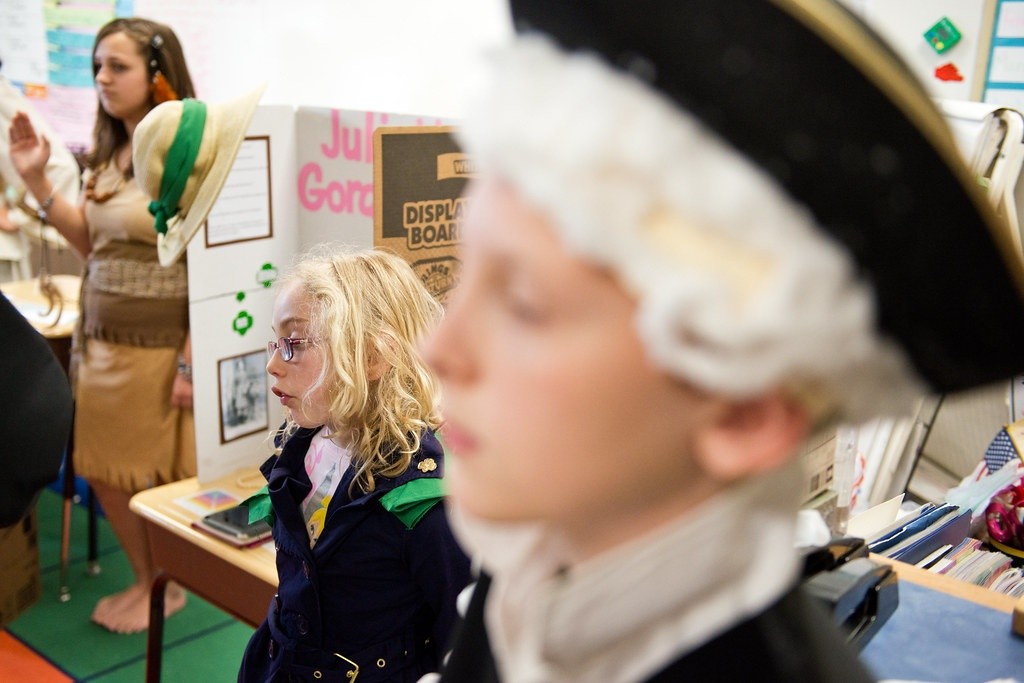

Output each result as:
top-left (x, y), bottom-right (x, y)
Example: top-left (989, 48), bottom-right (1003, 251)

top-left (267, 337), bottom-right (312, 362)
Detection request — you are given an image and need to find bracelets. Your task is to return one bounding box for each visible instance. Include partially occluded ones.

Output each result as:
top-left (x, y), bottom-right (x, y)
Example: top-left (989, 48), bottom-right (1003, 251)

top-left (38, 185), bottom-right (59, 208)
top-left (174, 356), bottom-right (193, 377)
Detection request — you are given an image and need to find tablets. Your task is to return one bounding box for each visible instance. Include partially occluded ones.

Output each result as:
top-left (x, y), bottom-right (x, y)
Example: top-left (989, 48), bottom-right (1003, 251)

top-left (203, 505), bottom-right (271, 538)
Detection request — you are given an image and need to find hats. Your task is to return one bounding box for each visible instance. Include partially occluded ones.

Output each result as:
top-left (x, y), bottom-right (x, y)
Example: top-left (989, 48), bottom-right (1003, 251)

top-left (133, 84), bottom-right (268, 268)
top-left (509, 0), bottom-right (1024, 401)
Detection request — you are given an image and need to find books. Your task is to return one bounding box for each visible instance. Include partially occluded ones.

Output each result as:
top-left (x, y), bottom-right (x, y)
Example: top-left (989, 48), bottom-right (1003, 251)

top-left (190, 503), bottom-right (275, 549)
top-left (870, 500), bottom-right (1024, 602)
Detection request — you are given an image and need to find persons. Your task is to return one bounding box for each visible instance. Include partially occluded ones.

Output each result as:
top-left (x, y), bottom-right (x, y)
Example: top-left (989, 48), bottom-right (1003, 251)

top-left (235, 245), bottom-right (445, 683)
top-left (0, 69), bottom-right (87, 372)
top-left (7, 18), bottom-right (198, 634)
top-left (299, 0), bottom-right (1024, 683)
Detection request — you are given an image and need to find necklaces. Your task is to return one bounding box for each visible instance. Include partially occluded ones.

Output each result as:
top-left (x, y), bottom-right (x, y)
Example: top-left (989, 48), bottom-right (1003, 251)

top-left (84, 149), bottom-right (137, 206)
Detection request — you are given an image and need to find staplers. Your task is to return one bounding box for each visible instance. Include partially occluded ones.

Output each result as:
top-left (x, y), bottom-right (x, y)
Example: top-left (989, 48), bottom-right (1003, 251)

top-left (800, 557), bottom-right (900, 657)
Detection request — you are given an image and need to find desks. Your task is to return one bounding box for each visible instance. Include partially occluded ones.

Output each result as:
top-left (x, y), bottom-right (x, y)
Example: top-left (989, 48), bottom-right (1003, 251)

top-left (128, 477), bottom-right (279, 683)
top-left (850, 551), bottom-right (1024, 683)
top-left (0, 275), bottom-right (101, 601)
